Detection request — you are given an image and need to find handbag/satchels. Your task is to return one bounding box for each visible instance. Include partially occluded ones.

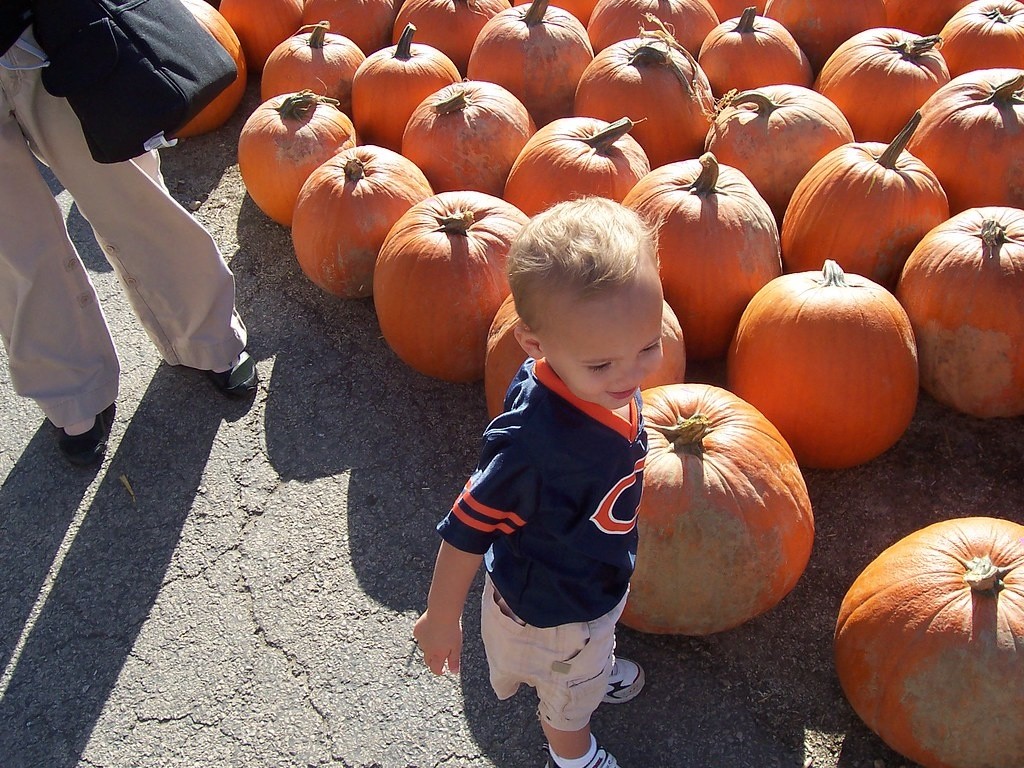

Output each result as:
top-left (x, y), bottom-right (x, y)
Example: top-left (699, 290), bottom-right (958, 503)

top-left (31, 0), bottom-right (238, 164)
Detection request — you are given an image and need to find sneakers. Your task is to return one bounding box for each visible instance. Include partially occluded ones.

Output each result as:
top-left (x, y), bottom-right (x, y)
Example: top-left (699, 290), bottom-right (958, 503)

top-left (57, 413), bottom-right (106, 465)
top-left (542, 742), bottom-right (621, 768)
top-left (205, 351), bottom-right (257, 398)
top-left (601, 655), bottom-right (646, 704)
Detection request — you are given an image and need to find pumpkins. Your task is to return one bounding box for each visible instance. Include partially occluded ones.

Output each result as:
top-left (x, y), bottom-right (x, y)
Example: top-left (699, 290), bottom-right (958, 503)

top-left (833, 517), bottom-right (1024, 768)
top-left (617, 384), bottom-right (814, 638)
top-left (164, 0), bottom-right (1024, 468)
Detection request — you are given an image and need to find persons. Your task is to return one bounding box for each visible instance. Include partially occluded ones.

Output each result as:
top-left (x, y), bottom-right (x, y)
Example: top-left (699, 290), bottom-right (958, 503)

top-left (0, 0), bottom-right (255, 459)
top-left (413, 196), bottom-right (663, 768)
